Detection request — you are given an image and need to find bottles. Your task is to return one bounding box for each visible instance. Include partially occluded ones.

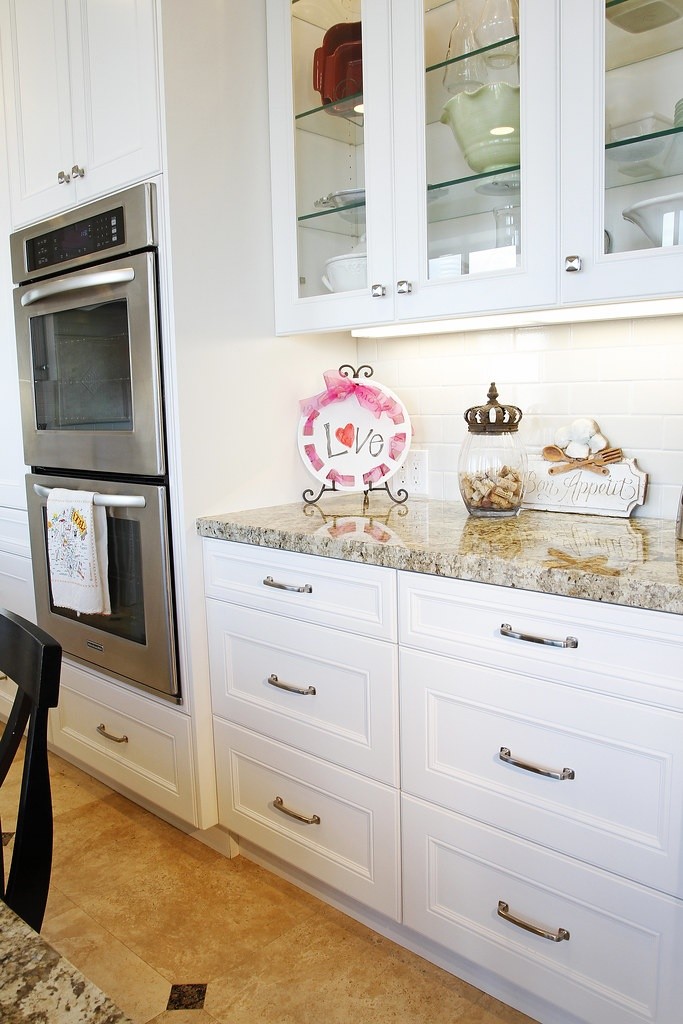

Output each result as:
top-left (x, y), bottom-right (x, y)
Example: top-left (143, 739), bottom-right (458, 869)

top-left (473, 0), bottom-right (519, 68)
top-left (492, 206), bottom-right (520, 257)
top-left (441, 2), bottom-right (488, 94)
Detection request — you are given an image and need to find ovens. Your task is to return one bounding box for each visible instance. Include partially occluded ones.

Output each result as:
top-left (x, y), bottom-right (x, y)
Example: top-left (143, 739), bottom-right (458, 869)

top-left (20, 248), bottom-right (180, 700)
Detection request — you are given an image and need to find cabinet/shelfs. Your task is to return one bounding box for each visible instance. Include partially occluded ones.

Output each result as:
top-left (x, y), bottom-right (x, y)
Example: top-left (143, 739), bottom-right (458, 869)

top-left (1, 501), bottom-right (239, 860)
top-left (264, 0), bottom-right (683, 338)
top-left (0, 0), bottom-right (163, 237)
top-left (198, 535), bottom-right (683, 1023)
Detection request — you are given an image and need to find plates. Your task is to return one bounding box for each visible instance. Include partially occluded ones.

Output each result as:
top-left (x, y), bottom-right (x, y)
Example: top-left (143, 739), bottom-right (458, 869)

top-left (673, 99), bottom-right (683, 128)
top-left (314, 184), bottom-right (449, 223)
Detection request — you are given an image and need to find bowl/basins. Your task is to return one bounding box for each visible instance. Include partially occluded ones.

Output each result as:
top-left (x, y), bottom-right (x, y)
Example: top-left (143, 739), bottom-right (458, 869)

top-left (320, 233), bottom-right (368, 293)
